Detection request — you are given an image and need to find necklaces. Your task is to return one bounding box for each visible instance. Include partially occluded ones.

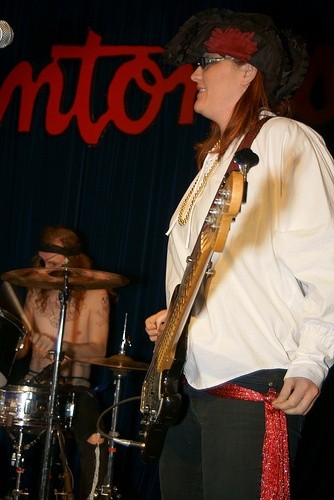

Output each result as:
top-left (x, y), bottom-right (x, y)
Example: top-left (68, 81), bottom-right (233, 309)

top-left (178, 139), bottom-right (221, 225)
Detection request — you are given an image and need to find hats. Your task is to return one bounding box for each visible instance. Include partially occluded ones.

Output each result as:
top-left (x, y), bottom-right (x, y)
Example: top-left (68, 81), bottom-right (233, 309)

top-left (206, 13), bottom-right (309, 103)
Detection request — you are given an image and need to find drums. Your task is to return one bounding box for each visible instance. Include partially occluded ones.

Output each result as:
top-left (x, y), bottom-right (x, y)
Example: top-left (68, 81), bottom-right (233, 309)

top-left (0, 384), bottom-right (95, 430)
top-left (0, 308), bottom-right (28, 388)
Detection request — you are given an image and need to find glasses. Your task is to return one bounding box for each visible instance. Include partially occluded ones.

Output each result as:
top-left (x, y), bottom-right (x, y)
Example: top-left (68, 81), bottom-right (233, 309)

top-left (191, 55), bottom-right (239, 73)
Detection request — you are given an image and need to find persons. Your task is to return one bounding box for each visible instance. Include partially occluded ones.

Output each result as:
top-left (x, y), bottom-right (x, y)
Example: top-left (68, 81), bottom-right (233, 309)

top-left (0, 227), bottom-right (110, 500)
top-left (144, 8), bottom-right (334, 500)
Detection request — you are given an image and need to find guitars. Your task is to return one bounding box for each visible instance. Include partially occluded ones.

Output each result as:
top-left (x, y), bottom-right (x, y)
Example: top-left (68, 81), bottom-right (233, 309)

top-left (140, 148), bottom-right (258, 458)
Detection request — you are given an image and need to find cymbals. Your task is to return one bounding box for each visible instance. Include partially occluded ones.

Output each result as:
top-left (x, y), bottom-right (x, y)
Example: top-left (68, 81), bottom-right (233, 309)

top-left (1, 266), bottom-right (127, 290)
top-left (73, 353), bottom-right (149, 372)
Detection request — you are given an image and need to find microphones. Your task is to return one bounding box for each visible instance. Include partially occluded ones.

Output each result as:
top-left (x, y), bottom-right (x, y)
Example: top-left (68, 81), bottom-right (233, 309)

top-left (112, 438), bottom-right (145, 448)
top-left (30, 363), bottom-right (55, 385)
top-left (0, 20), bottom-right (14, 49)
